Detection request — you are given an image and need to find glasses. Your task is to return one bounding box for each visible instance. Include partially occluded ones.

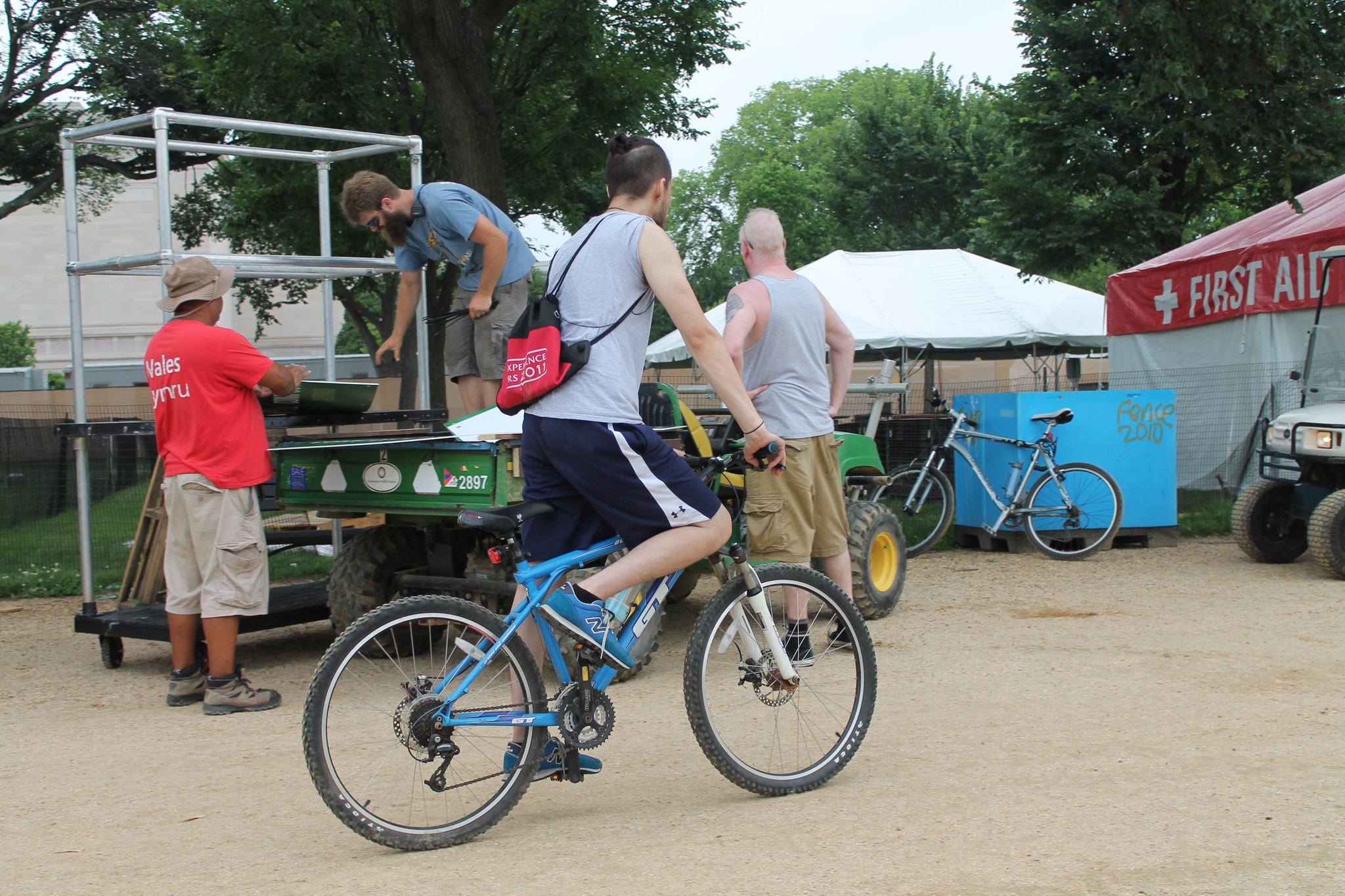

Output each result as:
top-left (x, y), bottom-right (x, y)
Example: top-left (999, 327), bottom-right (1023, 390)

top-left (366, 209), bottom-right (381, 227)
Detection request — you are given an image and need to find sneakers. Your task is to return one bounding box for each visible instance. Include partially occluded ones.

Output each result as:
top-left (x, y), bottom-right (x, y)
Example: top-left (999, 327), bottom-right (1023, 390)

top-left (202, 663), bottom-right (282, 714)
top-left (758, 624), bottom-right (815, 666)
top-left (501, 730), bottom-right (602, 782)
top-left (826, 620), bottom-right (853, 650)
top-left (537, 581), bottom-right (635, 670)
top-left (166, 655), bottom-right (207, 706)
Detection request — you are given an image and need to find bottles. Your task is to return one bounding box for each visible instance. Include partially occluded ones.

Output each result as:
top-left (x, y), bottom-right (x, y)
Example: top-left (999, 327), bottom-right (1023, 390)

top-left (603, 581), bottom-right (644, 635)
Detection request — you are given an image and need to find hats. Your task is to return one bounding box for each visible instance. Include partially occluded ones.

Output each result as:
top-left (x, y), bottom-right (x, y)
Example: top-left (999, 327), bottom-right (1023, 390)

top-left (154, 255), bottom-right (235, 311)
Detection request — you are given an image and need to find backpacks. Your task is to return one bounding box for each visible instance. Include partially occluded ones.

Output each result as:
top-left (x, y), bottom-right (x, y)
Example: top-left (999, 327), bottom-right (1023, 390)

top-left (494, 211), bottom-right (653, 417)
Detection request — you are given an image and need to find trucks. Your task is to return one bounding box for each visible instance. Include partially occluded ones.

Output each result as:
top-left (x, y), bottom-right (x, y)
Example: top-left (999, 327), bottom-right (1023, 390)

top-left (271, 382), bottom-right (906, 660)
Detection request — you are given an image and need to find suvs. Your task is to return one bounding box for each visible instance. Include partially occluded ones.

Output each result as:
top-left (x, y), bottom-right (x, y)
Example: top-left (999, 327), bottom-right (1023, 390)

top-left (1230, 245), bottom-right (1345, 581)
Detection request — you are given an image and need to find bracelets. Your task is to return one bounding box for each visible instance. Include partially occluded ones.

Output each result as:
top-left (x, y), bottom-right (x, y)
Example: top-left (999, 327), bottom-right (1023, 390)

top-left (742, 421), bottom-right (764, 435)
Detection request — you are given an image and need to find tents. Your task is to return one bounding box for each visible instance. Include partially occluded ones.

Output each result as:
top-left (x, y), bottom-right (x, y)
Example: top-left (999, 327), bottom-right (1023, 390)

top-left (1100, 169), bottom-right (1343, 491)
top-left (641, 249), bottom-right (1113, 414)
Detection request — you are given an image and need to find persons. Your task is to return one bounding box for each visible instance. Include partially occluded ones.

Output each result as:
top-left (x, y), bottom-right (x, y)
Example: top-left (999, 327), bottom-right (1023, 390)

top-left (340, 170), bottom-right (537, 412)
top-left (723, 206), bottom-right (857, 665)
top-left (143, 256), bottom-right (312, 715)
top-left (499, 131), bottom-right (786, 787)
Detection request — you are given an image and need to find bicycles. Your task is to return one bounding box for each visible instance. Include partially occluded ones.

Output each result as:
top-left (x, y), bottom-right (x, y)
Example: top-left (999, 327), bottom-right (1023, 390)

top-left (868, 386), bottom-right (1125, 561)
top-left (301, 440), bottom-right (880, 853)
